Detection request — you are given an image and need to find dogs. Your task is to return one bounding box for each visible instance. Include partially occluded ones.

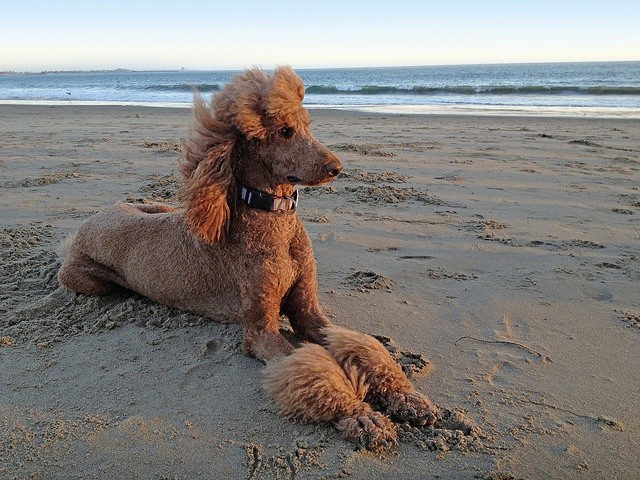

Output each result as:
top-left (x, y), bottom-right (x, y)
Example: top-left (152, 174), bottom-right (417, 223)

top-left (57, 64), bottom-right (474, 452)
